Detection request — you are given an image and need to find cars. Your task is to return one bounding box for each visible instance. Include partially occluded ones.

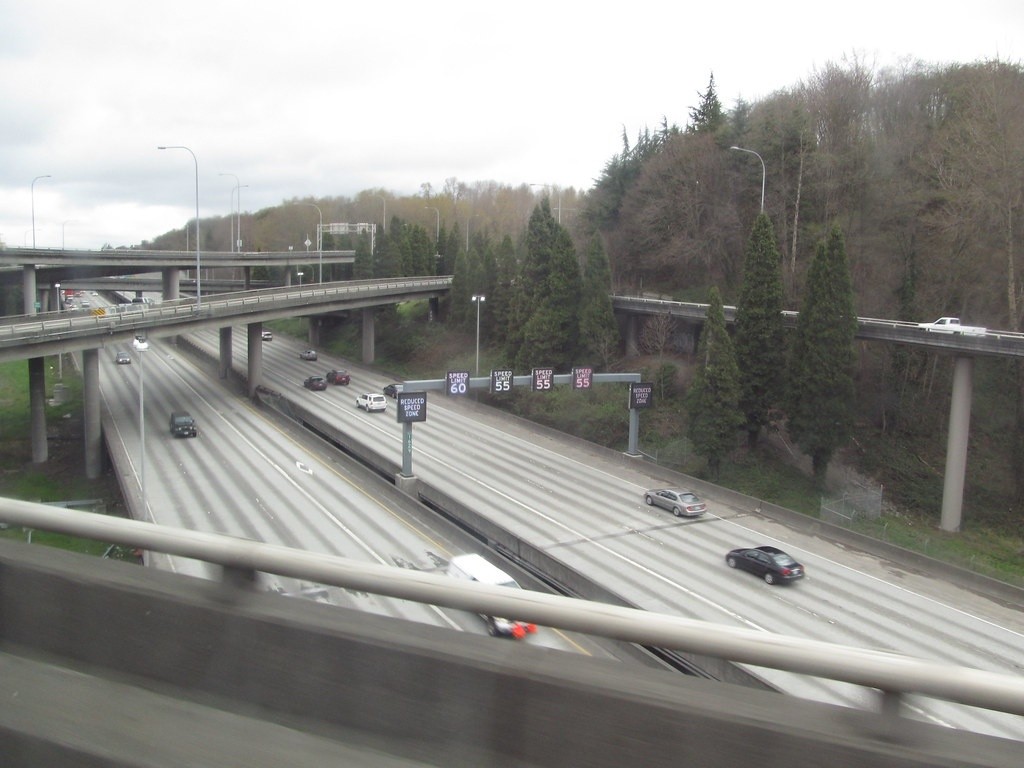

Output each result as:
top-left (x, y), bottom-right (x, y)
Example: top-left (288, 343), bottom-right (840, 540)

top-left (115, 351), bottom-right (131, 364)
top-left (64, 290), bottom-right (99, 312)
top-left (304, 375), bottom-right (327, 391)
top-left (300, 350), bottom-right (318, 361)
top-left (725, 545), bottom-right (806, 585)
top-left (169, 411), bottom-right (197, 438)
top-left (261, 329), bottom-right (275, 341)
top-left (326, 369), bottom-right (351, 386)
top-left (383, 383), bottom-right (403, 399)
top-left (643, 486), bottom-right (708, 517)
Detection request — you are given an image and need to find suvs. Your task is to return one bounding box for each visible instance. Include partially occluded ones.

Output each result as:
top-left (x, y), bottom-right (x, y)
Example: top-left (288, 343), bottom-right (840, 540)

top-left (356, 393), bottom-right (387, 413)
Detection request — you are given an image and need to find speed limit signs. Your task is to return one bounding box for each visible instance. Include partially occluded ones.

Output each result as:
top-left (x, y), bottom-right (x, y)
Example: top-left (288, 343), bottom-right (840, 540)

top-left (532, 367), bottom-right (554, 393)
top-left (490, 369), bottom-right (513, 393)
top-left (572, 368), bottom-right (593, 390)
top-left (444, 371), bottom-right (470, 399)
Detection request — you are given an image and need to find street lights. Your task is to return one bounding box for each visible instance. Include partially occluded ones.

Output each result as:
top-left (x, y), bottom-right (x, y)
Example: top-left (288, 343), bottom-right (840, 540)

top-left (218, 173), bottom-right (240, 252)
top-left (529, 183), bottom-right (561, 224)
top-left (132, 334), bottom-right (150, 524)
top-left (230, 184), bottom-right (249, 252)
top-left (424, 206), bottom-right (440, 257)
top-left (471, 295), bottom-right (486, 378)
top-left (729, 144), bottom-right (766, 216)
top-left (157, 145), bottom-right (202, 311)
top-left (293, 202), bottom-right (322, 283)
top-left (297, 272), bottom-right (304, 298)
top-left (54, 283), bottom-right (61, 314)
top-left (466, 214), bottom-right (479, 252)
top-left (30, 174), bottom-right (51, 249)
top-left (368, 193), bottom-right (386, 231)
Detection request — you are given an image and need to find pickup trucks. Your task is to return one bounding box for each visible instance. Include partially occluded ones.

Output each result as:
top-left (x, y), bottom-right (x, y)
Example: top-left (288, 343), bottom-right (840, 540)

top-left (924, 316), bottom-right (986, 336)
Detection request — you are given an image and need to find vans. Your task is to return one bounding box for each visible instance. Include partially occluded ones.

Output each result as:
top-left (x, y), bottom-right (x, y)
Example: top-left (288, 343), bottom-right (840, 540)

top-left (445, 552), bottom-right (538, 640)
